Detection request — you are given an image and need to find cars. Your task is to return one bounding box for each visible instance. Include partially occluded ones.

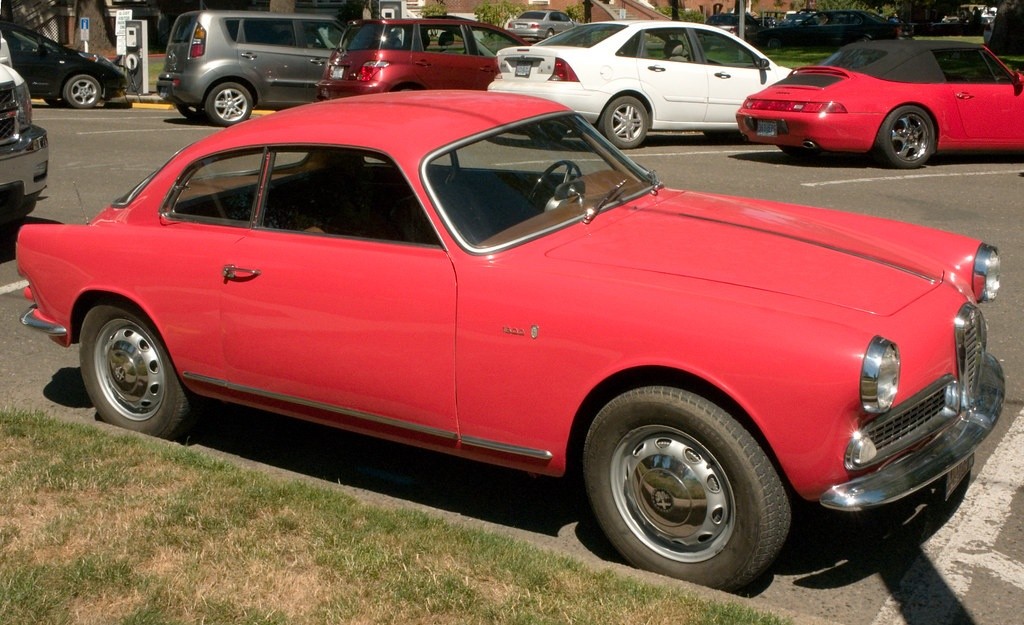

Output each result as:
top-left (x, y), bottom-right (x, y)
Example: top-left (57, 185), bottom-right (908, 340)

top-left (703, 8), bottom-right (907, 50)
top-left (939, 3), bottom-right (999, 45)
top-left (487, 19), bottom-right (797, 150)
top-left (0, 20), bottom-right (128, 109)
top-left (0, 31), bottom-right (50, 225)
top-left (505, 9), bottom-right (578, 41)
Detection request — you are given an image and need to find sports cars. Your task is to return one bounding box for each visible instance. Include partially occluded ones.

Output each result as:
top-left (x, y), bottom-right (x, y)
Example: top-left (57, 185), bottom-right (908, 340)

top-left (15, 90), bottom-right (1006, 595)
top-left (736, 39), bottom-right (1024, 169)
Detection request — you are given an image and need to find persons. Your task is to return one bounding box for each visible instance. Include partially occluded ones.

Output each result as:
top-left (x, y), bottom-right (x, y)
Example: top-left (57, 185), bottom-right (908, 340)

top-left (961, 7), bottom-right (973, 36)
top-left (973, 6), bottom-right (981, 36)
top-left (941, 15), bottom-right (950, 23)
top-left (878, 10), bottom-right (908, 38)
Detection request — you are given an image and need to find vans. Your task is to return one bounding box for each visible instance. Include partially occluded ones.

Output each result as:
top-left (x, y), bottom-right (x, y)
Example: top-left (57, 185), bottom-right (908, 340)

top-left (156, 10), bottom-right (363, 127)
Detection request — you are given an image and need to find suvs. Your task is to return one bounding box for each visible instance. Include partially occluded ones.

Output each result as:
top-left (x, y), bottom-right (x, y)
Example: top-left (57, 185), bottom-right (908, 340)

top-left (315, 15), bottom-right (533, 102)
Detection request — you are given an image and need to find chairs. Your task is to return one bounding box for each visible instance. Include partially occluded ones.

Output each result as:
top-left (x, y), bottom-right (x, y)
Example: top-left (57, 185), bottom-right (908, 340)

top-left (662, 42), bottom-right (688, 64)
top-left (391, 196), bottom-right (441, 245)
top-left (438, 32), bottom-right (456, 54)
